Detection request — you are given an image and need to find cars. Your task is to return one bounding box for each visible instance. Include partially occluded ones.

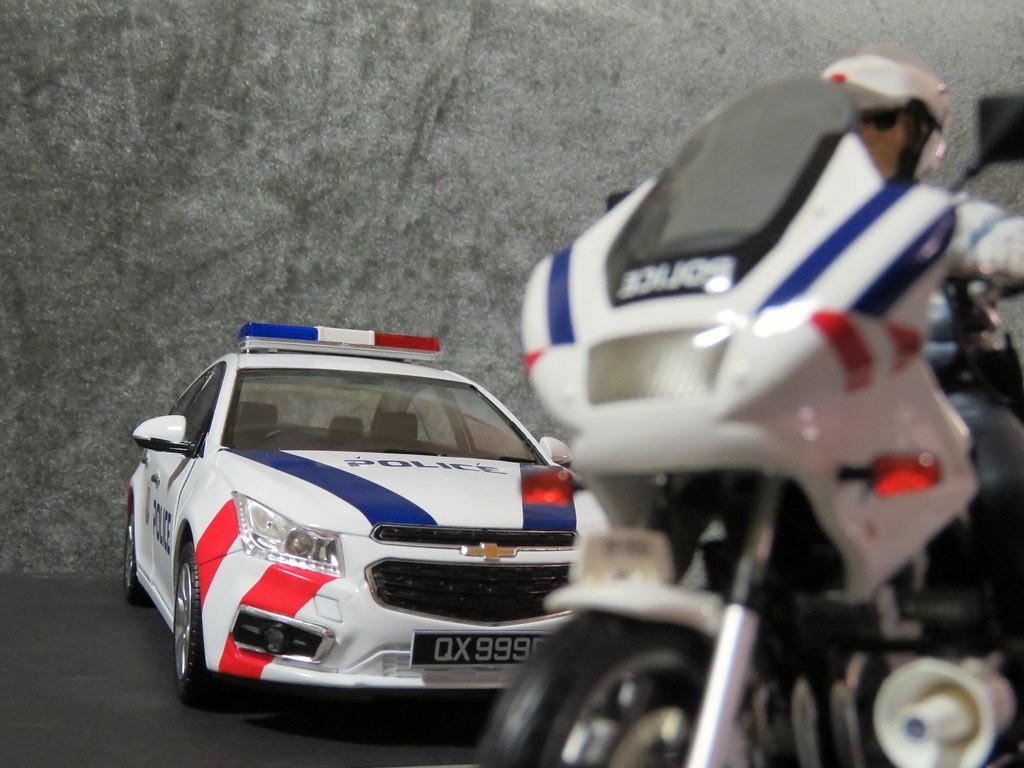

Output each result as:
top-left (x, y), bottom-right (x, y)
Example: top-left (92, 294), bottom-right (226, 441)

top-left (116, 321), bottom-right (582, 702)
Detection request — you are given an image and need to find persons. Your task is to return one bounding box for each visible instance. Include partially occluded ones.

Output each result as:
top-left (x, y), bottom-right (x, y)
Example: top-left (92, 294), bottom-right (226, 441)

top-left (821, 55), bottom-right (1024, 285)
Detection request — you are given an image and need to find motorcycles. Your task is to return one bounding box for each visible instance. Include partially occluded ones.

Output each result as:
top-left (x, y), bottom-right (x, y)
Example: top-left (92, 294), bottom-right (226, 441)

top-left (469, 49), bottom-right (1023, 768)
